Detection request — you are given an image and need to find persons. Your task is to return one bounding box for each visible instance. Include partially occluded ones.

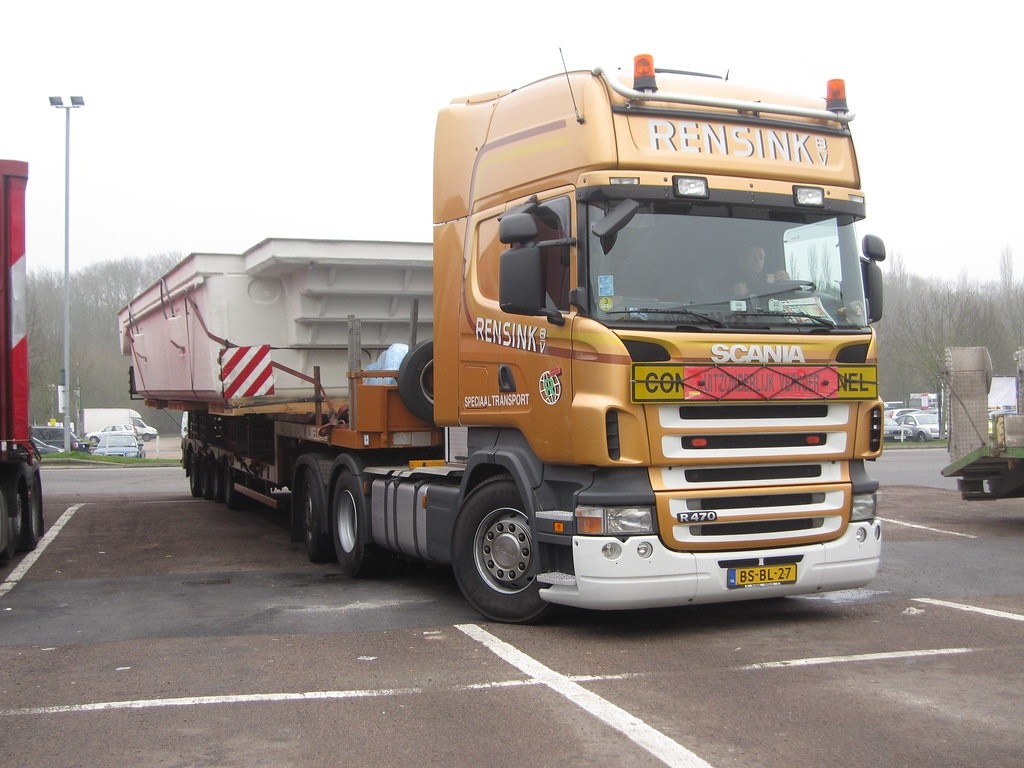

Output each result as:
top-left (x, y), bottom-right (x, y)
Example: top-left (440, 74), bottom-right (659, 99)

top-left (733, 245), bottom-right (789, 295)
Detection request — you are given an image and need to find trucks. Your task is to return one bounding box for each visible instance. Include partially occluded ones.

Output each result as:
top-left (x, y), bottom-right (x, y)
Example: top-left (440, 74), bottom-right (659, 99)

top-left (83, 408), bottom-right (162, 440)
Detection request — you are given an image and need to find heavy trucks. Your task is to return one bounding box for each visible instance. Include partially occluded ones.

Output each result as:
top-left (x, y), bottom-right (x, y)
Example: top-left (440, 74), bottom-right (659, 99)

top-left (0, 156), bottom-right (45, 562)
top-left (116, 50), bottom-right (889, 628)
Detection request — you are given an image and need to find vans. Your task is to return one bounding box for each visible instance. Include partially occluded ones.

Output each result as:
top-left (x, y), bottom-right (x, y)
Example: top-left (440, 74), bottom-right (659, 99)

top-left (32, 427), bottom-right (80, 451)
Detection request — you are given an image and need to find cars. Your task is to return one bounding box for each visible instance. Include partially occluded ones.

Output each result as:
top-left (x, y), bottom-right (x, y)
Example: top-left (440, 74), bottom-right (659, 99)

top-left (882, 401), bottom-right (939, 419)
top-left (93, 434), bottom-right (146, 459)
top-left (884, 417), bottom-right (913, 444)
top-left (897, 412), bottom-right (948, 441)
top-left (33, 437), bottom-right (61, 454)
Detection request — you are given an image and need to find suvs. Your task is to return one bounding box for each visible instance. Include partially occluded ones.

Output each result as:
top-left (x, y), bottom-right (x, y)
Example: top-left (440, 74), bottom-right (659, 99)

top-left (84, 425), bottom-right (139, 447)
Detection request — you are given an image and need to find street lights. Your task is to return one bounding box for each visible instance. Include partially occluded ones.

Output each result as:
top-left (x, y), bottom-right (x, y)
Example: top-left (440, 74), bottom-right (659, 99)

top-left (50, 96), bottom-right (85, 451)
